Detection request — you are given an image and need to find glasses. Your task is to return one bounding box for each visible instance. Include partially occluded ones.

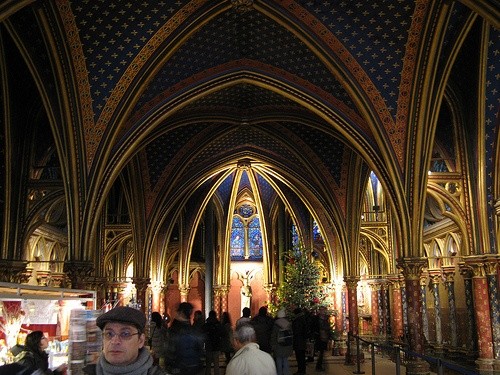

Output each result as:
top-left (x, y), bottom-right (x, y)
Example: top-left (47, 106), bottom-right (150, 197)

top-left (102, 328), bottom-right (141, 339)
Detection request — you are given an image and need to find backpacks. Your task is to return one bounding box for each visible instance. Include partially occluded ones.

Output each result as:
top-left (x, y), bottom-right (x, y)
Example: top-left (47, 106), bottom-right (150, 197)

top-left (277, 328), bottom-right (294, 346)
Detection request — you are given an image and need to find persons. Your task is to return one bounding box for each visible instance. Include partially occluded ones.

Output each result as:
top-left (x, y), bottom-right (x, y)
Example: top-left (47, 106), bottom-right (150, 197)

top-left (201, 310), bottom-right (227, 375)
top-left (165, 302), bottom-right (201, 375)
top-left (236, 307), bottom-right (252, 326)
top-left (221, 312), bottom-right (233, 362)
top-left (225, 322), bottom-right (277, 375)
top-left (311, 306), bottom-right (330, 371)
top-left (271, 310), bottom-right (294, 375)
top-left (22, 331), bottom-right (67, 375)
top-left (82, 306), bottom-right (168, 375)
top-left (301, 308), bottom-right (314, 362)
top-left (192, 311), bottom-right (204, 356)
top-left (150, 312), bottom-right (167, 369)
top-left (252, 307), bottom-right (268, 323)
top-left (292, 308), bottom-right (307, 375)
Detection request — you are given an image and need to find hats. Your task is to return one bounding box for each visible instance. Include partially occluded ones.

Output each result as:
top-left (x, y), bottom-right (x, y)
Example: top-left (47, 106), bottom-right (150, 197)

top-left (96, 306), bottom-right (146, 327)
